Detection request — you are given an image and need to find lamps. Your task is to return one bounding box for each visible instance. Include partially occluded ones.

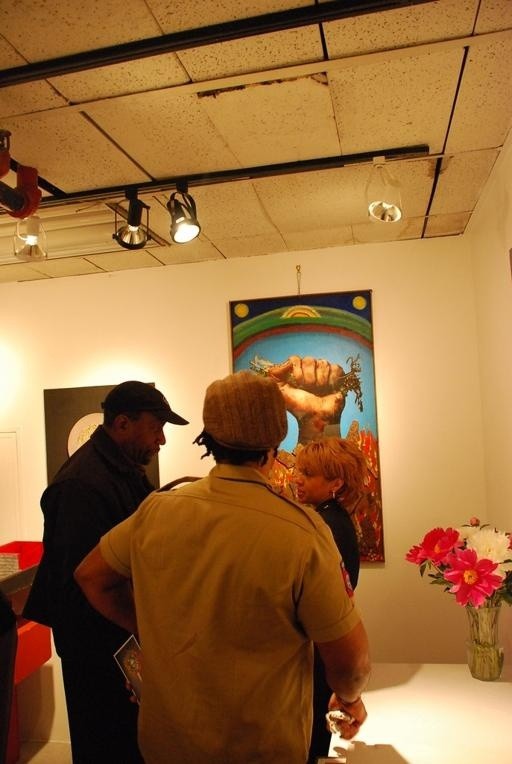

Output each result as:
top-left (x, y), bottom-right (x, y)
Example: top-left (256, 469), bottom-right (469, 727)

top-left (363, 144), bottom-right (404, 223)
top-left (112, 184), bottom-right (152, 250)
top-left (165, 174), bottom-right (201, 243)
top-left (12, 212), bottom-right (47, 262)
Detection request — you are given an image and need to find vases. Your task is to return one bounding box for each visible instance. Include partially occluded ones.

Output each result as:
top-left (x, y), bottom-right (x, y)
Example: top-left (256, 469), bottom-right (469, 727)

top-left (467, 605), bottom-right (504, 681)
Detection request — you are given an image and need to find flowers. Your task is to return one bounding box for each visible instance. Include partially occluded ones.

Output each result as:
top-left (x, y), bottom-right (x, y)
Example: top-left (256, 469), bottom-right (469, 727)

top-left (406, 515), bottom-right (512, 610)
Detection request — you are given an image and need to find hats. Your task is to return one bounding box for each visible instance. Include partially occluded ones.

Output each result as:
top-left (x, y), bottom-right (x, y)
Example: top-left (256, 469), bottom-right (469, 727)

top-left (203, 370), bottom-right (289, 451)
top-left (100, 380), bottom-right (189, 425)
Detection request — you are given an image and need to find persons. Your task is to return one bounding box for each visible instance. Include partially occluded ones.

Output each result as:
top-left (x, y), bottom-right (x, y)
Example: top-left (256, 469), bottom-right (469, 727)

top-left (0, 589), bottom-right (18, 763)
top-left (74, 369), bottom-right (371, 764)
top-left (295, 438), bottom-right (368, 764)
top-left (269, 356), bottom-right (345, 445)
top-left (21, 381), bottom-right (188, 764)
